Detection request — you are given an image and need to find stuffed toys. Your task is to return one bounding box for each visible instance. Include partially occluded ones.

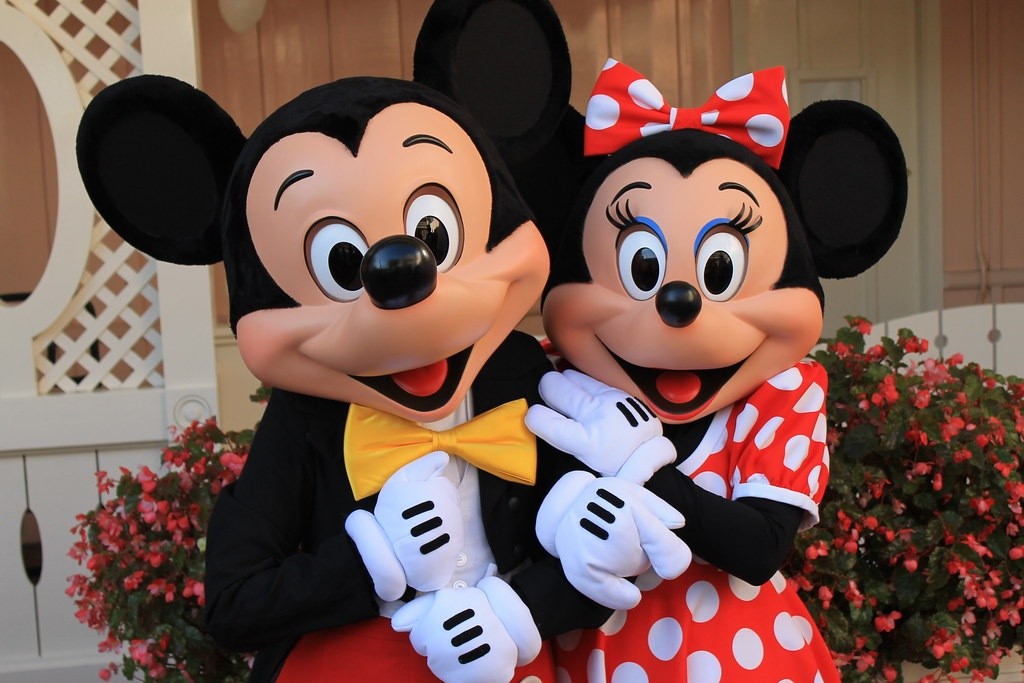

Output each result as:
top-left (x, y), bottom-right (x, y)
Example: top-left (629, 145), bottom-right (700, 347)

top-left (492, 56), bottom-right (911, 683)
top-left (74, 0), bottom-right (612, 683)
